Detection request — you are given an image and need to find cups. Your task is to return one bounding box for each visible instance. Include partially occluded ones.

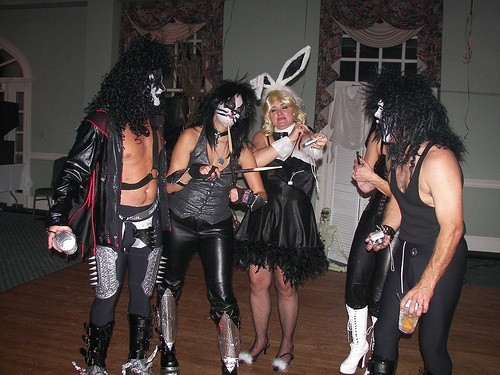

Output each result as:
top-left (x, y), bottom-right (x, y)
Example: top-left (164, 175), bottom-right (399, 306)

top-left (53, 230), bottom-right (78, 255)
top-left (398, 300), bottom-right (420, 334)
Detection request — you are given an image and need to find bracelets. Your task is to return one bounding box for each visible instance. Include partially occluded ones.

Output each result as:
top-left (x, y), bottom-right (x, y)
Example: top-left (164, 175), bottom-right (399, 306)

top-left (377, 225), bottom-right (396, 237)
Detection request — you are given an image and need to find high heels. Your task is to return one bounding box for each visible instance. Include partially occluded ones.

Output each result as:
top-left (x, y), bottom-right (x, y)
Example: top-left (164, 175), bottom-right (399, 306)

top-left (239, 336), bottom-right (270, 364)
top-left (272, 342), bottom-right (294, 371)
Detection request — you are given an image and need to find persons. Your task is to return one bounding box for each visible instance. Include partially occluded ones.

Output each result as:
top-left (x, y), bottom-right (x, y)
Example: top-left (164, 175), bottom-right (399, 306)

top-left (235, 90), bottom-right (329, 371)
top-left (155, 78), bottom-right (267, 375)
top-left (47, 42), bottom-right (172, 375)
top-left (364, 74), bottom-right (469, 375)
top-left (340, 129), bottom-right (400, 375)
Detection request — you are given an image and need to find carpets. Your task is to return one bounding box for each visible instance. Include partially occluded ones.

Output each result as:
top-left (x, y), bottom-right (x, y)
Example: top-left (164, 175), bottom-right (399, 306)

top-left (0, 207), bottom-right (91, 294)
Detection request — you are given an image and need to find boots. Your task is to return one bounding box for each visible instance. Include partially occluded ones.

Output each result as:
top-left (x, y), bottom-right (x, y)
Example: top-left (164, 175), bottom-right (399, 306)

top-left (363, 315), bottom-right (378, 375)
top-left (155, 326), bottom-right (179, 371)
top-left (71, 320), bottom-right (115, 375)
top-left (122, 313), bottom-right (158, 375)
top-left (340, 304), bottom-right (369, 374)
top-left (220, 356), bottom-right (238, 375)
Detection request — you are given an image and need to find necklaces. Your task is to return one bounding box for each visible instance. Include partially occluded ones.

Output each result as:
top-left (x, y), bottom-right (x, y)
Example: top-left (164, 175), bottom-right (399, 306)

top-left (215, 140), bottom-right (228, 166)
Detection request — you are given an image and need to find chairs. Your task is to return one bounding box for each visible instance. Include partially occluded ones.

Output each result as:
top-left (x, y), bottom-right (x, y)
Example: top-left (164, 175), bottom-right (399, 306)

top-left (31, 156), bottom-right (68, 220)
